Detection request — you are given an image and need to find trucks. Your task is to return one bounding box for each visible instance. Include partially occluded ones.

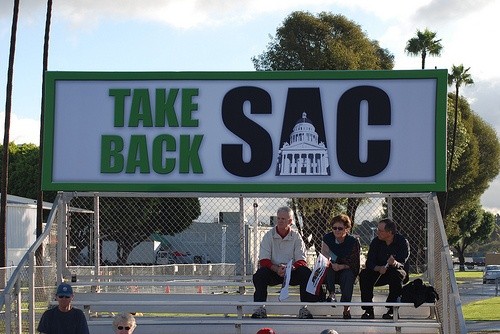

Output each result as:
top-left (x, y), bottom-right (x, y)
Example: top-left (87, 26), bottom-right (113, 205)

top-left (100, 239), bottom-right (172, 266)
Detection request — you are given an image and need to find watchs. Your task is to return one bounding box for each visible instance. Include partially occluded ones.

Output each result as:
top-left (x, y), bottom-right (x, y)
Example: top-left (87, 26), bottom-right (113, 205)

top-left (291, 264), bottom-right (296, 271)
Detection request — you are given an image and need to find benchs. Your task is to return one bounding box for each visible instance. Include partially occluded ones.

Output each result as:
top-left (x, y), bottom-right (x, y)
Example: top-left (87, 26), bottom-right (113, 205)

top-left (44, 272), bottom-right (442, 334)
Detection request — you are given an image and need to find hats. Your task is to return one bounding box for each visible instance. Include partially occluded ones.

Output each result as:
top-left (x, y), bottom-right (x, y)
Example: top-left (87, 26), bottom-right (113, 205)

top-left (56, 283), bottom-right (72, 296)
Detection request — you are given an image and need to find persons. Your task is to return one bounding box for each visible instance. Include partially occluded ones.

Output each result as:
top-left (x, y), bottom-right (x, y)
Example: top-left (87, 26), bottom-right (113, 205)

top-left (37, 281), bottom-right (89, 333)
top-left (112, 311), bottom-right (137, 333)
top-left (251, 205), bottom-right (321, 319)
top-left (321, 211), bottom-right (361, 319)
top-left (359, 218), bottom-right (410, 319)
top-left (194, 254), bottom-right (215, 267)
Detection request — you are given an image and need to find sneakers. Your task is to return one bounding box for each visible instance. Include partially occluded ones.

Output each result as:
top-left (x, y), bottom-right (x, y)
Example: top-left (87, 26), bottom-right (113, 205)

top-left (299, 307), bottom-right (312, 318)
top-left (251, 308), bottom-right (266, 318)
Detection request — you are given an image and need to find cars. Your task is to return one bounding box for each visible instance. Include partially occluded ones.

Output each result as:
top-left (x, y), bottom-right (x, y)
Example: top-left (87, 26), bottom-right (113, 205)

top-left (482, 263), bottom-right (500, 284)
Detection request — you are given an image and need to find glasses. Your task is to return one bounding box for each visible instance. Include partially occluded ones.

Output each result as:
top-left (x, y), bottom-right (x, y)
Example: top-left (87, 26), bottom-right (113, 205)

top-left (117, 326), bottom-right (131, 330)
top-left (332, 226), bottom-right (346, 231)
top-left (59, 296), bottom-right (70, 298)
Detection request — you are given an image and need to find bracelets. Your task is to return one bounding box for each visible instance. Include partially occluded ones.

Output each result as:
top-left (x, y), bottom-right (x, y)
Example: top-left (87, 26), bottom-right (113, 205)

top-left (343, 264), bottom-right (347, 270)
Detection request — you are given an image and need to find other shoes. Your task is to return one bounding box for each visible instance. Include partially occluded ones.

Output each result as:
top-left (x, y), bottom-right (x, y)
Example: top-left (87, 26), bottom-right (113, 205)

top-left (361, 310), bottom-right (374, 319)
top-left (342, 312), bottom-right (352, 319)
top-left (327, 296), bottom-right (337, 308)
top-left (382, 311), bottom-right (399, 318)
